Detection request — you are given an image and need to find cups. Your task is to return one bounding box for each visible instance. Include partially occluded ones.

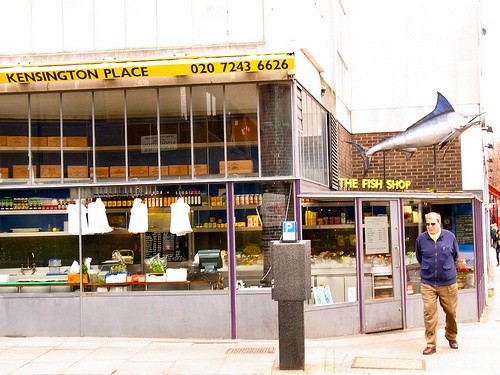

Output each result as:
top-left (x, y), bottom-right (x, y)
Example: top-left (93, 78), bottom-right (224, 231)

top-left (316, 217), bottom-right (341, 224)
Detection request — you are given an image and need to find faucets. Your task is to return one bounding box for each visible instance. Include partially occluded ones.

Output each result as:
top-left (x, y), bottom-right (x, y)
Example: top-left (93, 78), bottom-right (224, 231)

top-left (27, 252), bottom-right (35, 268)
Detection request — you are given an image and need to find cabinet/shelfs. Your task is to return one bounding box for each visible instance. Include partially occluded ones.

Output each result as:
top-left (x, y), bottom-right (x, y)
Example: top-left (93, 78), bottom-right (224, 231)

top-left (0, 140), bottom-right (263, 261)
top-left (301, 223), bottom-right (424, 302)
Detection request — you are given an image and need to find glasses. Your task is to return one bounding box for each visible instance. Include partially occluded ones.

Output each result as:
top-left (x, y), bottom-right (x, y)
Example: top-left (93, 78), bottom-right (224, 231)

top-left (425, 222), bottom-right (437, 227)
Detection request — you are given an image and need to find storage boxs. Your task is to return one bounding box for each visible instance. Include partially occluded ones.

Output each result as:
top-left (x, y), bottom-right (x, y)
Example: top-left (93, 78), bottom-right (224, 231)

top-left (219, 160), bottom-right (253, 174)
top-left (12, 165), bottom-right (37, 178)
top-left (0, 168), bottom-right (10, 178)
top-left (68, 274), bottom-right (89, 284)
top-left (0, 134), bottom-right (89, 147)
top-left (90, 164), bottom-right (208, 177)
top-left (102, 267), bottom-right (188, 283)
top-left (67, 165), bottom-right (87, 177)
top-left (40, 164), bottom-right (61, 177)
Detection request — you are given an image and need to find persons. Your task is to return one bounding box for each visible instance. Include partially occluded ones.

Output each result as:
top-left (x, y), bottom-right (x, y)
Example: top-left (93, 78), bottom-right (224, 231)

top-left (415, 212), bottom-right (459, 355)
top-left (491, 222), bottom-right (498, 247)
top-left (493, 230), bottom-right (500, 266)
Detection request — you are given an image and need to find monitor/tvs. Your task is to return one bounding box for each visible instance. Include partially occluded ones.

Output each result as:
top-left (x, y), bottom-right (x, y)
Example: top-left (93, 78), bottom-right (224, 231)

top-left (198, 250), bottom-right (223, 273)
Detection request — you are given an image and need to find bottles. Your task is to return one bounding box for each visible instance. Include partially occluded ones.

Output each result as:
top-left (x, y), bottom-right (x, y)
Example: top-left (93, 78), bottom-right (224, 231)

top-left (0, 197), bottom-right (86, 210)
top-left (85, 190), bottom-right (201, 208)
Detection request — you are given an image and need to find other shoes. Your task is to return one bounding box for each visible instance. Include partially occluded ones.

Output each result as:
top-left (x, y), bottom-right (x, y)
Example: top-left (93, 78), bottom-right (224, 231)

top-left (444, 335), bottom-right (459, 349)
top-left (422, 347), bottom-right (437, 354)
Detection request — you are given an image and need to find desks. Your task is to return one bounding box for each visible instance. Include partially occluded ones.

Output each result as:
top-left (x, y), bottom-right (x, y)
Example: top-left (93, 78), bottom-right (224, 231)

top-left (0, 281), bottom-right (213, 293)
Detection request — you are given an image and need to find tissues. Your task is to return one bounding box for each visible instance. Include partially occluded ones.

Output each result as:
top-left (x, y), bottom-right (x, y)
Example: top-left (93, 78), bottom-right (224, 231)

top-left (68, 260), bottom-right (89, 283)
